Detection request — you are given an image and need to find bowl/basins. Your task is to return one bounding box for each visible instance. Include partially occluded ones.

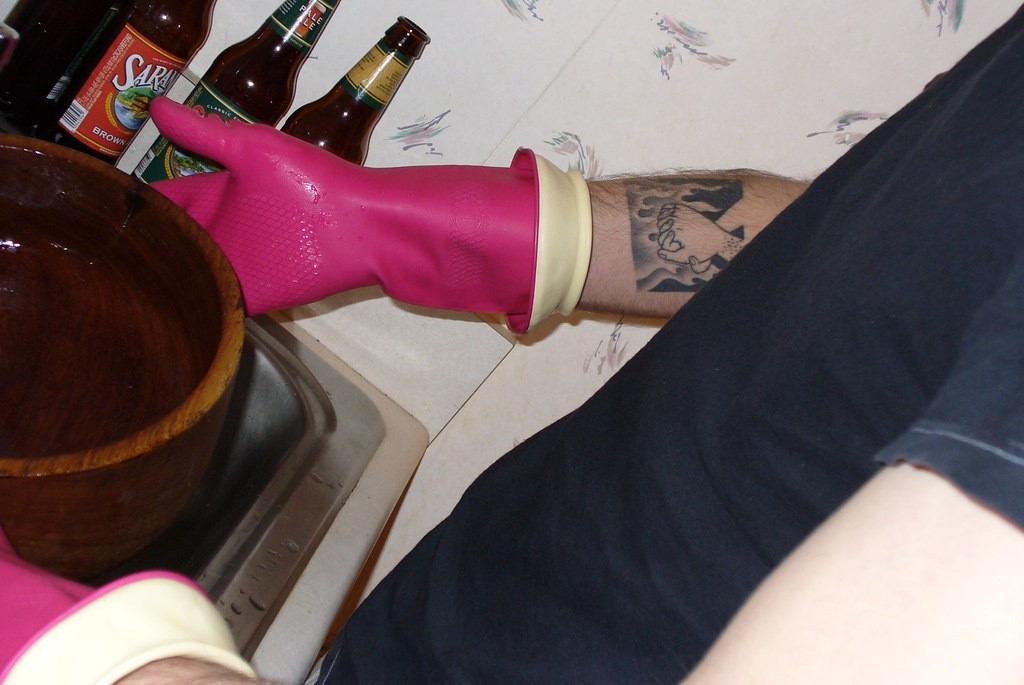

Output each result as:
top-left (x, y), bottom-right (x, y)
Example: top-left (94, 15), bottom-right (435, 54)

top-left (0, 136), bottom-right (243, 580)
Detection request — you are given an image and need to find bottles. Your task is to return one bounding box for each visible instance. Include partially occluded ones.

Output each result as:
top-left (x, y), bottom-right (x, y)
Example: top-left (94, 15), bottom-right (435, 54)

top-left (35, 0), bottom-right (217, 165)
top-left (281, 15), bottom-right (430, 166)
top-left (130, 0), bottom-right (340, 183)
top-left (0, 0), bottom-right (127, 136)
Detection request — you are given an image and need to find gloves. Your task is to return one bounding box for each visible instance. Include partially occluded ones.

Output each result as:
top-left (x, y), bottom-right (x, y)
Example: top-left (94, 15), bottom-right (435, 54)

top-left (146, 96), bottom-right (593, 335)
top-left (0, 528), bottom-right (258, 685)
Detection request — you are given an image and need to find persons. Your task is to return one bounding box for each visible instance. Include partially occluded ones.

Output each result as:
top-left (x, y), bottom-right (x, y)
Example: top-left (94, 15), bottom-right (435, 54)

top-left (2, 0), bottom-right (1022, 685)
